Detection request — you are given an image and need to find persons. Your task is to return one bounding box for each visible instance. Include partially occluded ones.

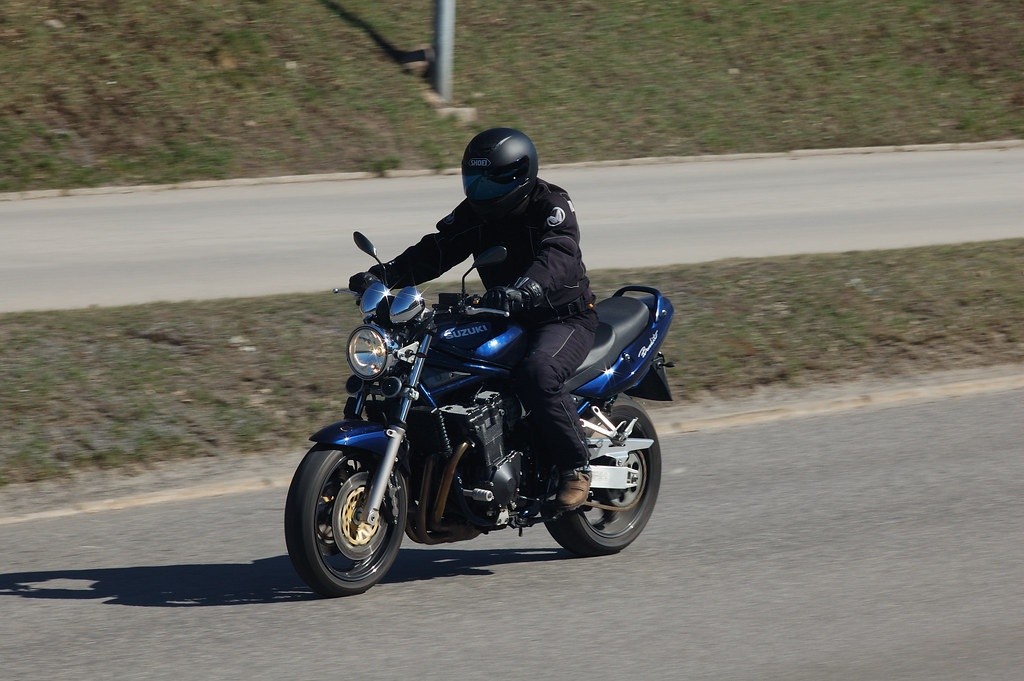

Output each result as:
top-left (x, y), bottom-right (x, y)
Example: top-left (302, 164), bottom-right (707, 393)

top-left (347, 126), bottom-right (602, 512)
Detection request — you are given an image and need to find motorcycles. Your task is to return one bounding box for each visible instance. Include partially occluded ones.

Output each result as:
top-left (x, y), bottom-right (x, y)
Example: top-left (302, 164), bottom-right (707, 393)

top-left (285, 232), bottom-right (674, 597)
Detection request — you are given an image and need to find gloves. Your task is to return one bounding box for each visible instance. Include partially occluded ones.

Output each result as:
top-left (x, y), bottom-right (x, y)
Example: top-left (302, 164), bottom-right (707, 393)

top-left (478, 275), bottom-right (544, 315)
top-left (348, 271), bottom-right (381, 296)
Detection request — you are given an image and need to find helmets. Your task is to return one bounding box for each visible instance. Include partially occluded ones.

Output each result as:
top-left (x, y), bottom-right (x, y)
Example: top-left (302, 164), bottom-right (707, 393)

top-left (461, 127), bottom-right (539, 216)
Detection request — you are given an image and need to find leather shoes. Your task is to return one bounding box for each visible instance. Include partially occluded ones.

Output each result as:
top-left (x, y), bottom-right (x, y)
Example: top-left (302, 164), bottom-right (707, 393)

top-left (554, 466), bottom-right (593, 513)
